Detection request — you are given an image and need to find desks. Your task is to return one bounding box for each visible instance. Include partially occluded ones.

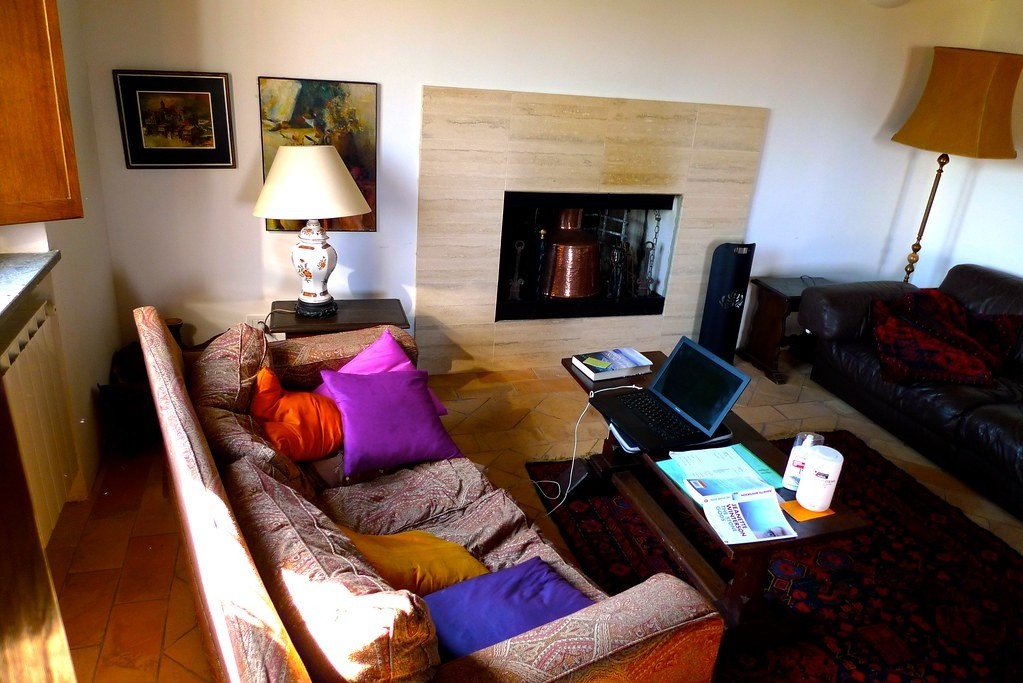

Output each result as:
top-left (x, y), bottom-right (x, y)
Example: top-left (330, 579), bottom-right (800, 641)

top-left (735, 277), bottom-right (835, 385)
top-left (268, 300), bottom-right (410, 339)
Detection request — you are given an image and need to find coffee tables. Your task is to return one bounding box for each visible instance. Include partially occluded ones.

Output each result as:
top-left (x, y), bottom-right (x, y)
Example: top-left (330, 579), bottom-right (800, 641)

top-left (561, 349), bottom-right (863, 637)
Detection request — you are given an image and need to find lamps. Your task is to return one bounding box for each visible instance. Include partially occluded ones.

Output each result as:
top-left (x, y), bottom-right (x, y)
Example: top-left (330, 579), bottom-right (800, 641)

top-left (254, 145), bottom-right (372, 317)
top-left (891, 45), bottom-right (1023, 282)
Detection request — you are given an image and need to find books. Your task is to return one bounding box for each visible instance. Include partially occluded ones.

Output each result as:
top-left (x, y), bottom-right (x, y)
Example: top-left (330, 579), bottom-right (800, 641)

top-left (656, 447), bottom-right (797, 544)
top-left (572, 347), bottom-right (652, 381)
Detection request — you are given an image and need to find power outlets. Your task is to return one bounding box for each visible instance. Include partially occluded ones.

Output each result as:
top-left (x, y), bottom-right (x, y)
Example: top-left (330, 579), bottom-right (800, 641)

top-left (246, 314), bottom-right (273, 333)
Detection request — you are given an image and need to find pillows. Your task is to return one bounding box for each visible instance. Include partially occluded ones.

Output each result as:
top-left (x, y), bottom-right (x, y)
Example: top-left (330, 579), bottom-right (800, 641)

top-left (250, 366), bottom-right (343, 463)
top-left (334, 523), bottom-right (492, 599)
top-left (422, 556), bottom-right (597, 658)
top-left (311, 329), bottom-right (448, 419)
top-left (319, 368), bottom-right (467, 480)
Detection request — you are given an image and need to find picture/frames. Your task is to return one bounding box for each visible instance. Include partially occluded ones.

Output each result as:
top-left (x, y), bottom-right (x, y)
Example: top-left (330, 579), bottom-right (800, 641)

top-left (112, 68), bottom-right (236, 169)
top-left (258, 76), bottom-right (377, 233)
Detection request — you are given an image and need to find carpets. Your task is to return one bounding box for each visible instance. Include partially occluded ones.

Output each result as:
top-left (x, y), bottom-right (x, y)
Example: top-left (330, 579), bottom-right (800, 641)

top-left (525, 429), bottom-right (1023, 683)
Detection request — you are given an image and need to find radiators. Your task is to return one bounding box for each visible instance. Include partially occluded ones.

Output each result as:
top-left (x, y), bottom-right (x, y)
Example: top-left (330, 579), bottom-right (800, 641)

top-left (0, 299), bottom-right (81, 556)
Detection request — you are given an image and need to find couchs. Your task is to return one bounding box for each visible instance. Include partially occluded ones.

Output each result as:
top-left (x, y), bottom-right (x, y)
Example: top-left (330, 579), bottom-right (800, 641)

top-left (132, 306), bottom-right (725, 682)
top-left (796, 265), bottom-right (1023, 526)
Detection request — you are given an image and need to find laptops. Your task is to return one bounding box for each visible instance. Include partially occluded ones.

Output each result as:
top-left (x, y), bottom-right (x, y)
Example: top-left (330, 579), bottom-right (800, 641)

top-left (587, 336), bottom-right (750, 454)
